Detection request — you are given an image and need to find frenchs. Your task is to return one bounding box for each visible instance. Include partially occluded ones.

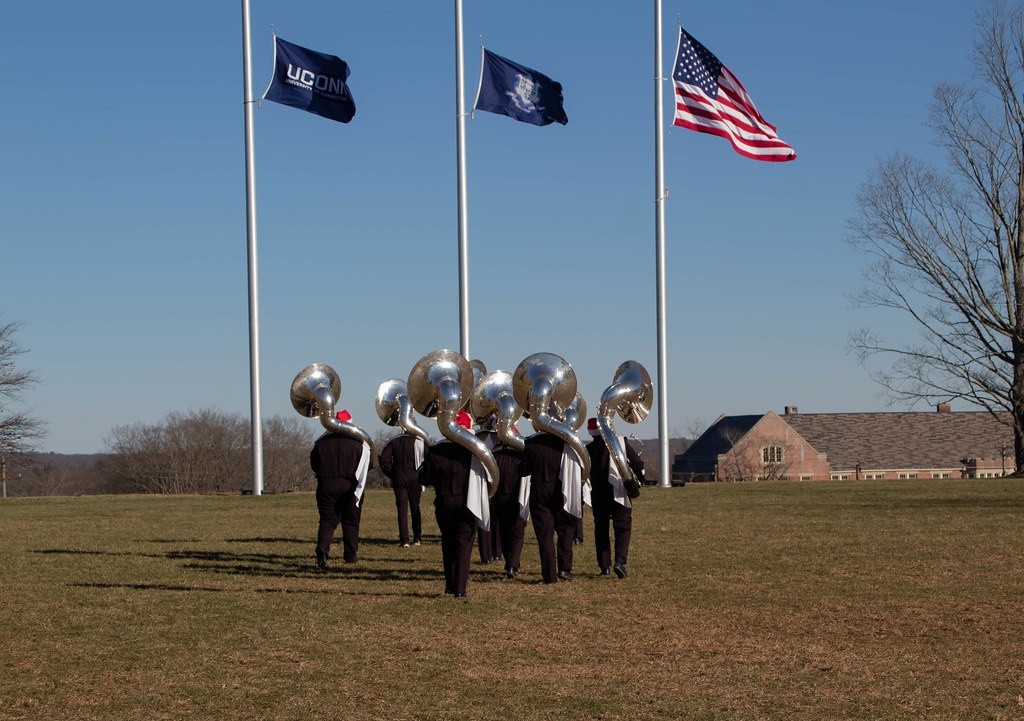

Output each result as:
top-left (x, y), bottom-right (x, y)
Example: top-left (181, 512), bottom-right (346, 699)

top-left (290, 363), bottom-right (375, 472)
top-left (512, 352), bottom-right (591, 489)
top-left (459, 358), bottom-right (588, 455)
top-left (376, 378), bottom-right (433, 479)
top-left (596, 359), bottom-right (654, 498)
top-left (406, 349), bottom-right (500, 499)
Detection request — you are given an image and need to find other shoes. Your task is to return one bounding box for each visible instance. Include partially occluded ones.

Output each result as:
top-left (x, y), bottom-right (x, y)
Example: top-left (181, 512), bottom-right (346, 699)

top-left (315, 547), bottom-right (327, 569)
top-left (485, 560), bottom-right (491, 564)
top-left (493, 557), bottom-right (502, 561)
top-left (507, 567), bottom-right (514, 579)
top-left (455, 593), bottom-right (466, 599)
top-left (404, 543), bottom-right (410, 548)
top-left (414, 541), bottom-right (420, 547)
top-left (558, 571), bottom-right (575, 580)
top-left (574, 538), bottom-right (583, 546)
top-left (600, 568), bottom-right (610, 575)
top-left (614, 563), bottom-right (627, 578)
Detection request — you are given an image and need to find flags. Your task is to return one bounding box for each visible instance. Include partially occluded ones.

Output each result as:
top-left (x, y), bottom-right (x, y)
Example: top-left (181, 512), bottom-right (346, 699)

top-left (262, 32), bottom-right (356, 123)
top-left (672, 26), bottom-right (798, 162)
top-left (473, 45), bottom-right (568, 126)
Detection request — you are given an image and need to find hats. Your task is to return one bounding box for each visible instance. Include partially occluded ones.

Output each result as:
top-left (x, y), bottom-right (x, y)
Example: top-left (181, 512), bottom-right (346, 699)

top-left (446, 410), bottom-right (473, 443)
top-left (336, 410), bottom-right (352, 423)
top-left (513, 427), bottom-right (519, 434)
top-left (587, 418), bottom-right (601, 436)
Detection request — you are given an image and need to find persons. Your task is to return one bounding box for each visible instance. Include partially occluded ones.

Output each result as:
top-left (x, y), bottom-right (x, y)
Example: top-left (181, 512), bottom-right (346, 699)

top-left (377, 422), bottom-right (432, 549)
top-left (477, 412), bottom-right (530, 580)
top-left (585, 418), bottom-right (646, 579)
top-left (309, 410), bottom-right (375, 568)
top-left (416, 409), bottom-right (490, 599)
top-left (525, 414), bottom-right (592, 586)
